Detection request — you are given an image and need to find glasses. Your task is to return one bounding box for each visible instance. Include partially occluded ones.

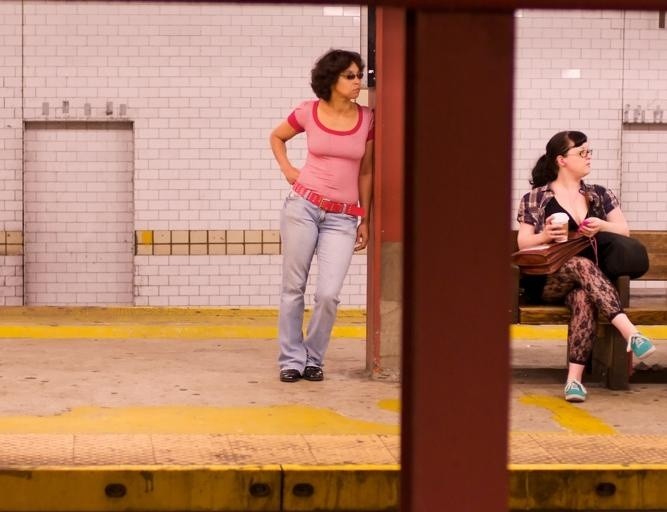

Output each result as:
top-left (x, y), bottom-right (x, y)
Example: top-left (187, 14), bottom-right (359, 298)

top-left (564, 149), bottom-right (593, 158)
top-left (338, 72), bottom-right (364, 80)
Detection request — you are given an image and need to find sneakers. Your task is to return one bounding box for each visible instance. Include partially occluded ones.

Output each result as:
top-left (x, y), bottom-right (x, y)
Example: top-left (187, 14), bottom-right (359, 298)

top-left (627, 332), bottom-right (656, 360)
top-left (563, 379), bottom-right (587, 402)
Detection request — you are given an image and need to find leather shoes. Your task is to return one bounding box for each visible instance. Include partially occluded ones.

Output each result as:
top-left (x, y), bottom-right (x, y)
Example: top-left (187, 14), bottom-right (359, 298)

top-left (303, 366), bottom-right (324, 381)
top-left (280, 368), bottom-right (301, 382)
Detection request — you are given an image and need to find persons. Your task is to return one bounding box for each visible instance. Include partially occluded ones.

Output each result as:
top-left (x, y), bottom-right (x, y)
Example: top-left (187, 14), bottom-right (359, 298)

top-left (514, 130), bottom-right (659, 404)
top-left (267, 47), bottom-right (377, 381)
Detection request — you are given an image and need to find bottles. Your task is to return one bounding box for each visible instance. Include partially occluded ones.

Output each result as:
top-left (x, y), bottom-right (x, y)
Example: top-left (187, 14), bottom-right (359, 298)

top-left (633, 105), bottom-right (646, 124)
top-left (624, 103), bottom-right (630, 122)
top-left (653, 105), bottom-right (665, 124)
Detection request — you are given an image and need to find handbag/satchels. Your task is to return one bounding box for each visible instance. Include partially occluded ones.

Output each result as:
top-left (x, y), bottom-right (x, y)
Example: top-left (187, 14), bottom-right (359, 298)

top-left (509, 236), bottom-right (594, 275)
top-left (578, 232), bottom-right (651, 281)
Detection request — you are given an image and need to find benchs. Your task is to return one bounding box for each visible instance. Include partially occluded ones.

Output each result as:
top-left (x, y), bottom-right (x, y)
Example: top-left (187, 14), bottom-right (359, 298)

top-left (506, 230), bottom-right (667, 391)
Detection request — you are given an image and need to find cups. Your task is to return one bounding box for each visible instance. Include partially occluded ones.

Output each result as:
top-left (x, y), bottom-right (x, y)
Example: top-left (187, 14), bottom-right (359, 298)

top-left (550, 212), bottom-right (570, 244)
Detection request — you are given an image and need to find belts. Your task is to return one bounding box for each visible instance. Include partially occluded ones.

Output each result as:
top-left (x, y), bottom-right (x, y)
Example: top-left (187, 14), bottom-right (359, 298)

top-left (291, 180), bottom-right (366, 218)
